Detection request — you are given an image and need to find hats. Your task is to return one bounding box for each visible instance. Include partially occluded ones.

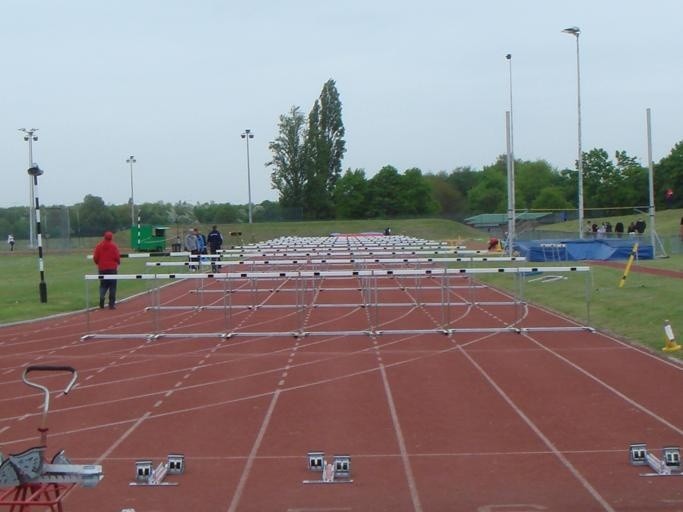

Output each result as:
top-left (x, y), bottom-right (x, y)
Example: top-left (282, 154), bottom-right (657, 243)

top-left (105, 231), bottom-right (113, 239)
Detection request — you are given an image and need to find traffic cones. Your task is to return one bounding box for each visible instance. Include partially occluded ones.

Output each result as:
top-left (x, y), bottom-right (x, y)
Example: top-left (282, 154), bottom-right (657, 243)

top-left (661, 319), bottom-right (682, 351)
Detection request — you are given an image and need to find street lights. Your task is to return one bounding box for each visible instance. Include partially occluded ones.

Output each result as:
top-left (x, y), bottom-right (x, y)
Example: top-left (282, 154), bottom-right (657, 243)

top-left (125, 155), bottom-right (137, 226)
top-left (560, 27), bottom-right (583, 239)
top-left (239, 128), bottom-right (254, 224)
top-left (18, 128), bottom-right (38, 247)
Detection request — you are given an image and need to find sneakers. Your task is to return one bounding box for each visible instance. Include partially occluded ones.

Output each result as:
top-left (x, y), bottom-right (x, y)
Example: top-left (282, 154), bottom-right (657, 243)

top-left (100, 298), bottom-right (105, 308)
top-left (109, 305), bottom-right (116, 309)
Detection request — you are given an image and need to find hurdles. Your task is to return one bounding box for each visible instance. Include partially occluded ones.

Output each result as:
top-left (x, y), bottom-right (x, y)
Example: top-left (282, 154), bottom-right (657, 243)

top-left (83, 235), bottom-right (594, 339)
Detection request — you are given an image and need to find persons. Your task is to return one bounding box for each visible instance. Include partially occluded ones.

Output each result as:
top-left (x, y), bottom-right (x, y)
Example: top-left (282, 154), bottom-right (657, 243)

top-left (487, 238), bottom-right (499, 249)
top-left (206, 225), bottom-right (223, 273)
top-left (584, 217), bottom-right (646, 239)
top-left (185, 228), bottom-right (210, 269)
top-left (94, 231), bottom-right (120, 310)
top-left (8, 233), bottom-right (14, 251)
top-left (384, 227), bottom-right (389, 235)
top-left (679, 217), bottom-right (683, 240)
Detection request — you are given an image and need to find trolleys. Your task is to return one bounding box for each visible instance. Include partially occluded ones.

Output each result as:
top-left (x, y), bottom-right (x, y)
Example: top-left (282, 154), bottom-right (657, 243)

top-left (0, 363), bottom-right (79, 511)
